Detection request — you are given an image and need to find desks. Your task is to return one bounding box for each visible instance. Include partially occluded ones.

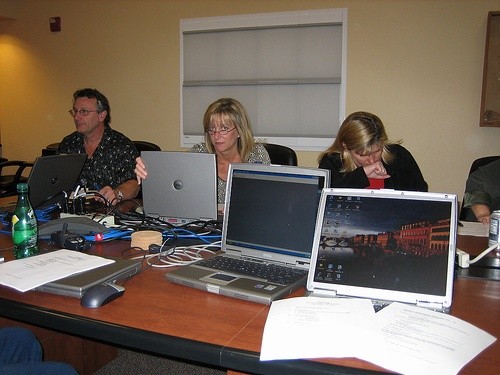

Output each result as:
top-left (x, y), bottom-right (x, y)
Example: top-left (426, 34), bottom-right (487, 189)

top-left (0, 194), bottom-right (500, 375)
top-left (0, 157), bottom-right (8, 162)
top-left (46, 143), bottom-right (60, 149)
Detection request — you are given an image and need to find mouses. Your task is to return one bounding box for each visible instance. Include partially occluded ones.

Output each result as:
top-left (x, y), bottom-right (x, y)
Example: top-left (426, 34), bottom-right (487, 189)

top-left (81, 282), bottom-right (126, 309)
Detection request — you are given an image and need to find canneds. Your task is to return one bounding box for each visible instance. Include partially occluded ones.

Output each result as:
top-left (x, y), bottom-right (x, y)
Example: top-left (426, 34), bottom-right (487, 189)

top-left (488, 210), bottom-right (500, 252)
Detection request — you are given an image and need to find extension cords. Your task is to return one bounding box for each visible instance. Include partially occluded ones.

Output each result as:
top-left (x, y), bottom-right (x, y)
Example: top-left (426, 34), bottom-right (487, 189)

top-left (59, 212), bottom-right (114, 226)
top-left (456, 248), bottom-right (470, 269)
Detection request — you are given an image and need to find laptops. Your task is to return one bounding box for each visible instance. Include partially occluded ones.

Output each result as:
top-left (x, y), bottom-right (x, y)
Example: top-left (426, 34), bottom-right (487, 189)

top-left (302, 187), bottom-right (459, 315)
top-left (2, 152), bottom-right (87, 214)
top-left (165, 163), bottom-right (331, 305)
top-left (141, 150), bottom-right (223, 224)
top-left (32, 255), bottom-right (142, 299)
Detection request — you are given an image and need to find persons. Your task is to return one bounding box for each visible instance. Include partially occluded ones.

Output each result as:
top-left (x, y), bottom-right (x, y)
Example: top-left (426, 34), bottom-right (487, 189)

top-left (463, 159), bottom-right (500, 224)
top-left (135, 98), bottom-right (271, 203)
top-left (57, 88), bottom-right (140, 205)
top-left (319, 111), bottom-right (429, 192)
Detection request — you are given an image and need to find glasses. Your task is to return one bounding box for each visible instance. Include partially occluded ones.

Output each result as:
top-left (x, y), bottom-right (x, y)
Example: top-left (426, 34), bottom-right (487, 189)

top-left (207, 126), bottom-right (237, 136)
top-left (68, 109), bottom-right (99, 116)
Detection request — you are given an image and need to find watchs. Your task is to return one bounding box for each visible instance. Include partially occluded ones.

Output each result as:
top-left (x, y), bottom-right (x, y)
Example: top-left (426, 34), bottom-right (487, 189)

top-left (114, 189), bottom-right (123, 203)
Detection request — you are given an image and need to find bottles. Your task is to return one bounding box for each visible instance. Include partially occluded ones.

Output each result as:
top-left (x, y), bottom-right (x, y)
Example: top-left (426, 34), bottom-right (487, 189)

top-left (12, 183), bottom-right (38, 259)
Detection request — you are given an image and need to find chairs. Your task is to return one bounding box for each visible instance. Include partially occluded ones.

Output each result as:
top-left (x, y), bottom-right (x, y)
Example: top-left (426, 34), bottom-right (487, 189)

top-left (264, 144), bottom-right (297, 166)
top-left (42, 148), bottom-right (57, 157)
top-left (132, 140), bottom-right (161, 200)
top-left (459, 156), bottom-right (500, 221)
top-left (0, 160), bottom-right (34, 197)
